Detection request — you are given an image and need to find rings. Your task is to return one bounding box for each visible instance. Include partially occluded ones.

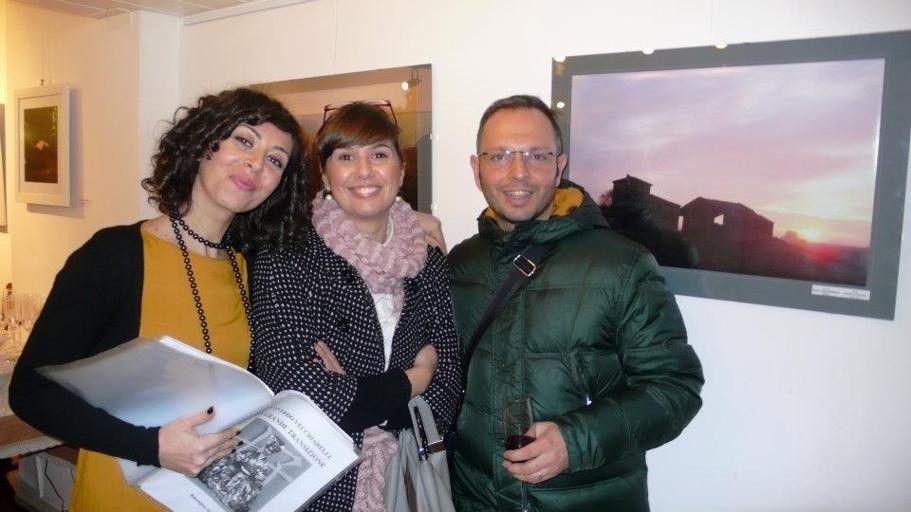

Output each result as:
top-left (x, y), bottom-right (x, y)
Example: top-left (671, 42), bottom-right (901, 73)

top-left (537, 470), bottom-right (543, 484)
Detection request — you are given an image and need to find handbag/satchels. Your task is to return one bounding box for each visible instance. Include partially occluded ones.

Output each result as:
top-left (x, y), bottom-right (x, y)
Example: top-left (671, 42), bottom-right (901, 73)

top-left (382, 394), bottom-right (457, 512)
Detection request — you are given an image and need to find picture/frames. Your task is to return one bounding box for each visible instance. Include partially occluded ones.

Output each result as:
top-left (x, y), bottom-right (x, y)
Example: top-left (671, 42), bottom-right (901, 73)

top-left (12, 83), bottom-right (71, 208)
top-left (550, 30), bottom-right (909, 319)
top-left (248, 64), bottom-right (429, 213)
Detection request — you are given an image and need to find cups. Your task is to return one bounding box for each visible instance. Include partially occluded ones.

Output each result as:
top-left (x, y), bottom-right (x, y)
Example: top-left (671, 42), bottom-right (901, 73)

top-left (2, 287), bottom-right (15, 323)
top-left (6, 294), bottom-right (19, 353)
top-left (14, 295), bottom-right (26, 347)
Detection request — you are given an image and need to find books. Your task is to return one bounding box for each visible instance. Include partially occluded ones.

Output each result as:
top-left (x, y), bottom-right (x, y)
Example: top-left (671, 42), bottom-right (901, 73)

top-left (37, 333), bottom-right (364, 511)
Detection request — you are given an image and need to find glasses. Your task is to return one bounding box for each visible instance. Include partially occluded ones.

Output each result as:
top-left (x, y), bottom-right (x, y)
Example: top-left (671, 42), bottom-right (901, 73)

top-left (479, 146), bottom-right (555, 168)
top-left (323, 98), bottom-right (399, 128)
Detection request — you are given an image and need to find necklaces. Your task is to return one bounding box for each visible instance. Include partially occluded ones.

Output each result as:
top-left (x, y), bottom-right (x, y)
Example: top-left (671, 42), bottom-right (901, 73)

top-left (164, 208), bottom-right (258, 376)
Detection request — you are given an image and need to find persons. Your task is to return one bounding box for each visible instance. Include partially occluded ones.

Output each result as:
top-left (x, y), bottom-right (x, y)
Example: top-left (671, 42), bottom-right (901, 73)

top-left (448, 94), bottom-right (705, 512)
top-left (249, 100), bottom-right (461, 512)
top-left (7, 86), bottom-right (309, 512)
